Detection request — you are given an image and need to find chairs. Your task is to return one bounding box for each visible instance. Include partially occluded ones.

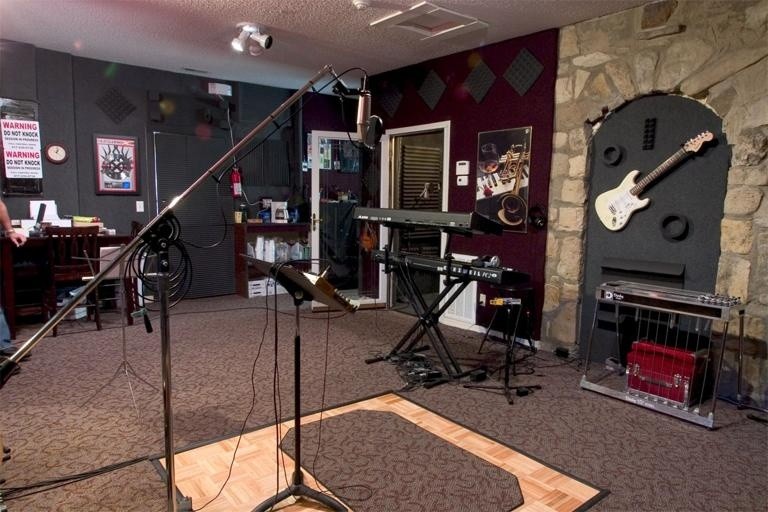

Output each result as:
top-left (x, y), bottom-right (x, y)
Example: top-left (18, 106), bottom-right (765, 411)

top-left (88, 220), bottom-right (146, 326)
top-left (40, 225), bottom-right (104, 338)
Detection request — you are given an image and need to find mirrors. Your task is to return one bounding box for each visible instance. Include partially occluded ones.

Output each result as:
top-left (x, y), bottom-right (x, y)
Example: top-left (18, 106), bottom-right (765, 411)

top-left (318, 136), bottom-right (381, 300)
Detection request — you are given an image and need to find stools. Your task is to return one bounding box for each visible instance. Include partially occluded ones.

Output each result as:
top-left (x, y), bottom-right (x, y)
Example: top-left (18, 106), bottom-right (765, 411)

top-left (477, 285), bottom-right (536, 356)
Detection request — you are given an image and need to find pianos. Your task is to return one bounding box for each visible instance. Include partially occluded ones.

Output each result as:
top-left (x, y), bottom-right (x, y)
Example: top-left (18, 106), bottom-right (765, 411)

top-left (371, 248), bottom-right (533, 285)
top-left (353, 204), bottom-right (503, 239)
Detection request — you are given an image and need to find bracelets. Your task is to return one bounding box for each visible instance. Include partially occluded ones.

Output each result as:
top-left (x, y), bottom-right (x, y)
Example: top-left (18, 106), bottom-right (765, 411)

top-left (5, 228), bottom-right (14, 235)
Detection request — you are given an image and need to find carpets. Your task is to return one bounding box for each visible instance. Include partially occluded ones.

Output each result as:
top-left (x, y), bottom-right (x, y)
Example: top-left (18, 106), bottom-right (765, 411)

top-left (276, 407), bottom-right (525, 512)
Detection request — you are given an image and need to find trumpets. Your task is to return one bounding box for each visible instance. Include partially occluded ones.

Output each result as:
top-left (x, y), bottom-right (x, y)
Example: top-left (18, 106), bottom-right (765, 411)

top-left (493, 128), bottom-right (530, 228)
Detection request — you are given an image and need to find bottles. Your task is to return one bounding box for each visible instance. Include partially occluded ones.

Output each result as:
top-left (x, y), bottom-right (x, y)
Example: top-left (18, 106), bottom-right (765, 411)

top-left (239, 201), bottom-right (248, 224)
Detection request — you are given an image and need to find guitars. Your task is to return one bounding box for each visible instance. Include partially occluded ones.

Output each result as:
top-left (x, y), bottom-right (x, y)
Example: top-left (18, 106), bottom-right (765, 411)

top-left (594, 127), bottom-right (715, 232)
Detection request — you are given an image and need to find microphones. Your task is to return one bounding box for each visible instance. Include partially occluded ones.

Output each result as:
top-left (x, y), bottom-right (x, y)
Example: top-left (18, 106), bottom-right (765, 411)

top-left (352, 87), bottom-right (383, 149)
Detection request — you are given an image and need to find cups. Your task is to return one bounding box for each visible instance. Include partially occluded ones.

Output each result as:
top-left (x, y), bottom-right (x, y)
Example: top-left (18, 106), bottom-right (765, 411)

top-left (234, 211), bottom-right (242, 224)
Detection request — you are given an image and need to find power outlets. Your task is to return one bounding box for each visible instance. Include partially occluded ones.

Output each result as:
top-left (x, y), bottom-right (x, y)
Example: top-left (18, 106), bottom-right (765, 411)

top-left (478, 292), bottom-right (487, 308)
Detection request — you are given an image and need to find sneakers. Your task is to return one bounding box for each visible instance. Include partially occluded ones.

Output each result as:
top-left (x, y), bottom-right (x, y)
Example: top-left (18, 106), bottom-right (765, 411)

top-left (0, 346), bottom-right (32, 374)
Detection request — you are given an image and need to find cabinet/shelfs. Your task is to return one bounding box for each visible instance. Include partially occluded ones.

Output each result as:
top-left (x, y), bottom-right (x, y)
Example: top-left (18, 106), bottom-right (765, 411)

top-left (232, 222), bottom-right (311, 299)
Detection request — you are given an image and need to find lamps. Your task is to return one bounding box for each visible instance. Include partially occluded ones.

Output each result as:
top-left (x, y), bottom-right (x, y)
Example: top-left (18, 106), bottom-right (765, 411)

top-left (230, 21), bottom-right (273, 57)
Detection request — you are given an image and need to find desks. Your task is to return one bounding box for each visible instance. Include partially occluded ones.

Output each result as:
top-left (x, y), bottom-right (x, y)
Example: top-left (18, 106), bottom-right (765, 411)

top-left (0, 231), bottom-right (137, 341)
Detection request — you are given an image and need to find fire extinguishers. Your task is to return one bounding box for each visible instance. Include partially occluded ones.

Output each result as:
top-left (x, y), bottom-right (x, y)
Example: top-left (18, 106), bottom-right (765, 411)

top-left (230, 167), bottom-right (242, 197)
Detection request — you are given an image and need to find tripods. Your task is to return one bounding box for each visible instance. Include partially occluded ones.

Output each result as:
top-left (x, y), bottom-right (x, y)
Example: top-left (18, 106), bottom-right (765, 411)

top-left (246, 298), bottom-right (348, 512)
top-left (78, 257), bottom-right (160, 422)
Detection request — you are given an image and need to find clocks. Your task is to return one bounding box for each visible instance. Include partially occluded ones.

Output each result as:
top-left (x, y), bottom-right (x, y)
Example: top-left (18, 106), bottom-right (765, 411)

top-left (44, 142), bottom-right (70, 165)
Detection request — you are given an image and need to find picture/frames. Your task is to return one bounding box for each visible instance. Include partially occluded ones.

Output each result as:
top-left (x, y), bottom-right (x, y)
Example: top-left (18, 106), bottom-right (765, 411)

top-left (473, 125), bottom-right (534, 235)
top-left (92, 132), bottom-right (142, 196)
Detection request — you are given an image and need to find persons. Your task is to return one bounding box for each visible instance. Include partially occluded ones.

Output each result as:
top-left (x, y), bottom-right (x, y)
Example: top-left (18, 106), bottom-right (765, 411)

top-left (0, 198), bottom-right (35, 360)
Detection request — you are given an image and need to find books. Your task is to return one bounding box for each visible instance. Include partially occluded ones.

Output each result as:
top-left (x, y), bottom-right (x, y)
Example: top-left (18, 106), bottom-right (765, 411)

top-left (11, 199), bottom-right (116, 238)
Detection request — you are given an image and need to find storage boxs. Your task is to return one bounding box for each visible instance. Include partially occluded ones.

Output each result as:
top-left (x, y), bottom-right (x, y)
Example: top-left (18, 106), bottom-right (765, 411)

top-left (625, 336), bottom-right (715, 411)
